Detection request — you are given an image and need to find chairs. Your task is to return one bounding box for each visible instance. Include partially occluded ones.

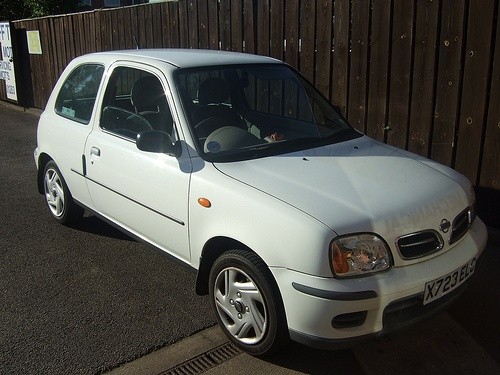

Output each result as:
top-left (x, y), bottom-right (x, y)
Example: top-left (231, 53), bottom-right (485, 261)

top-left (192, 79), bottom-right (243, 137)
top-left (124, 76), bottom-right (172, 135)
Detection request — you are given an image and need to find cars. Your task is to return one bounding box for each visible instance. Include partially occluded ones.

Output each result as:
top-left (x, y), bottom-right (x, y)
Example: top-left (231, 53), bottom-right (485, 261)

top-left (33, 46), bottom-right (490, 359)
